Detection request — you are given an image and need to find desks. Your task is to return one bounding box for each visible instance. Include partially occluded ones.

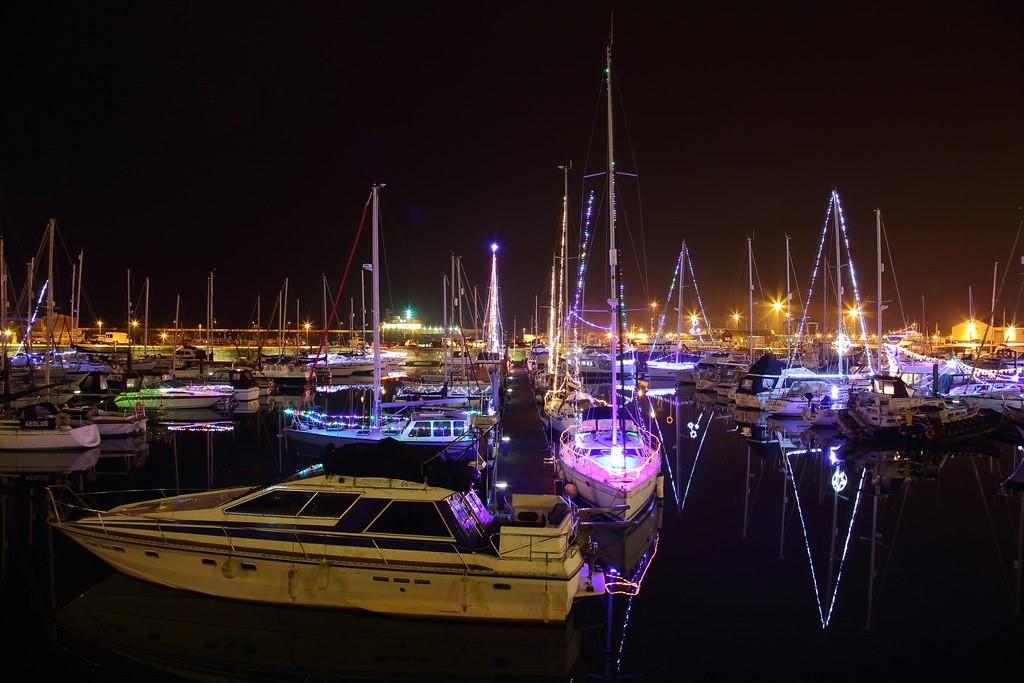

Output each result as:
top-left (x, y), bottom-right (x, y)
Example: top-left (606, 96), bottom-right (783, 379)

top-left (576, 432), bottom-right (644, 458)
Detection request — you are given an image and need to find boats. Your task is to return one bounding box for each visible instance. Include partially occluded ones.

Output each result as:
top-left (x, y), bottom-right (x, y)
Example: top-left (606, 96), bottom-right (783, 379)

top-left (0, 178), bottom-right (506, 450)
top-left (44, 399), bottom-right (629, 626)
top-left (647, 187), bottom-right (1024, 452)
top-left (506, 159), bottom-right (604, 442)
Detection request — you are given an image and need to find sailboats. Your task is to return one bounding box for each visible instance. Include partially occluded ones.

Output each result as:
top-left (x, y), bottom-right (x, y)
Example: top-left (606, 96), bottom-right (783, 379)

top-left (526, 0), bottom-right (665, 584)
